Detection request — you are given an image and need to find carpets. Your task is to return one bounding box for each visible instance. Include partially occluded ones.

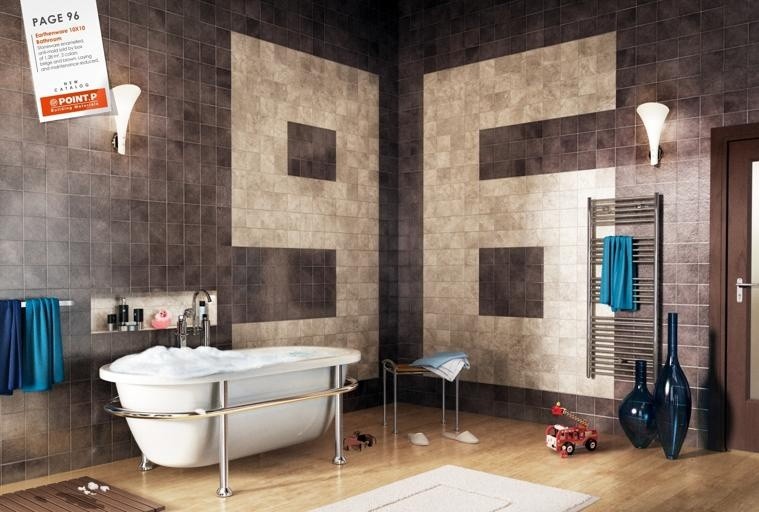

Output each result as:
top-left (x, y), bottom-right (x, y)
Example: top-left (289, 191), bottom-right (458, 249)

top-left (308, 463), bottom-right (600, 512)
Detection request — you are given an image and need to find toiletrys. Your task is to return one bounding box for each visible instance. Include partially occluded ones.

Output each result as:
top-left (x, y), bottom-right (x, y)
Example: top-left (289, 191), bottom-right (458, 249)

top-left (199, 300), bottom-right (206, 326)
top-left (198, 300), bottom-right (207, 321)
top-left (119, 298), bottom-right (129, 325)
top-left (202, 314), bottom-right (208, 327)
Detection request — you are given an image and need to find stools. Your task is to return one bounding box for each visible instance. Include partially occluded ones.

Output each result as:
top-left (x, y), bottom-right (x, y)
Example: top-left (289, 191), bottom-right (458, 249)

top-left (382, 358), bottom-right (460, 434)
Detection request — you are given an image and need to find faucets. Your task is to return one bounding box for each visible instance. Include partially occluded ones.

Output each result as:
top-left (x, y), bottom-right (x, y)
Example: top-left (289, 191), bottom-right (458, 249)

top-left (191, 289), bottom-right (212, 334)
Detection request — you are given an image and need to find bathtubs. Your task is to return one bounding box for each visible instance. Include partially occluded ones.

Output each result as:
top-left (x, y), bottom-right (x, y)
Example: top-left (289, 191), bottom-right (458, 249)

top-left (99, 344), bottom-right (362, 471)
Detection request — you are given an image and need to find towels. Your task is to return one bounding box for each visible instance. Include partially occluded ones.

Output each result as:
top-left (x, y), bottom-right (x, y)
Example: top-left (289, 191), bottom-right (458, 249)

top-left (22, 297), bottom-right (65, 392)
top-left (0, 300), bottom-right (22, 395)
top-left (600, 235), bottom-right (637, 312)
top-left (423, 359), bottom-right (470, 382)
top-left (408, 352), bottom-right (469, 369)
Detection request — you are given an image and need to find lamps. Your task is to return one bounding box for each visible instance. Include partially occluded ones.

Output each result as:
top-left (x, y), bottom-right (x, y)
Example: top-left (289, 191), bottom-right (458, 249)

top-left (109, 84), bottom-right (142, 156)
top-left (637, 101), bottom-right (670, 165)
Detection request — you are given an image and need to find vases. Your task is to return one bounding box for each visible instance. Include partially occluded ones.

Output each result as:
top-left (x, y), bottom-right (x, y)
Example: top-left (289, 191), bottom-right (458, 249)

top-left (653, 312), bottom-right (692, 460)
top-left (618, 360), bottom-right (658, 449)
